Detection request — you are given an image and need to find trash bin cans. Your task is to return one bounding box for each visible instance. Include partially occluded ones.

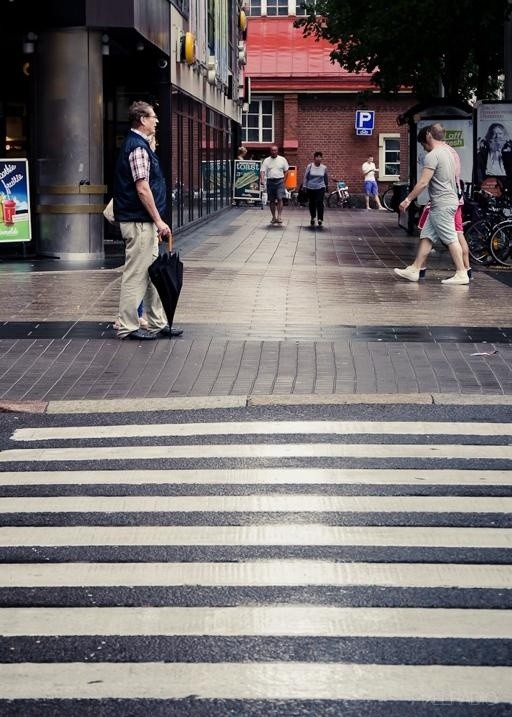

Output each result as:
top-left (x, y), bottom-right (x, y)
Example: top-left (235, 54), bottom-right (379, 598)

top-left (284, 166), bottom-right (298, 188)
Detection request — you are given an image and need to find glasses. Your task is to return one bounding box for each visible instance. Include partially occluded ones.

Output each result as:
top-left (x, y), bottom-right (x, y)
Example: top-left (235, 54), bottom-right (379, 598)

top-left (146, 114), bottom-right (158, 119)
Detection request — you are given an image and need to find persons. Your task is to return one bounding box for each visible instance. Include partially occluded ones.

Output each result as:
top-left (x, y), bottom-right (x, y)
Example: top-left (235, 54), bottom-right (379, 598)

top-left (475, 122), bottom-right (510, 179)
top-left (393, 122), bottom-right (471, 285)
top-left (236, 146), bottom-right (248, 160)
top-left (259, 145), bottom-right (289, 224)
top-left (411, 124), bottom-right (474, 281)
top-left (302, 151), bottom-right (330, 226)
top-left (361, 154), bottom-right (388, 211)
top-left (114, 99), bottom-right (184, 341)
top-left (112, 133), bottom-right (159, 333)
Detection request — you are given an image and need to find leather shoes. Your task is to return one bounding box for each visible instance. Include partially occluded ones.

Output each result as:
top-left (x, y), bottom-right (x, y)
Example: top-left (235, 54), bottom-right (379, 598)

top-left (120, 325), bottom-right (184, 339)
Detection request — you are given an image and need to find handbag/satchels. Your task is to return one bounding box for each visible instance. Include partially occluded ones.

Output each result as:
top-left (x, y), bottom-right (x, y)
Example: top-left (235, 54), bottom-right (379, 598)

top-left (296, 183), bottom-right (309, 203)
top-left (102, 196), bottom-right (116, 225)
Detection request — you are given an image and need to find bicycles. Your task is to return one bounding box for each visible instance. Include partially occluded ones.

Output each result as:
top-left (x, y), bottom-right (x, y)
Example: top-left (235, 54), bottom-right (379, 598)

top-left (463, 182), bottom-right (512, 268)
top-left (327, 179), bottom-right (353, 209)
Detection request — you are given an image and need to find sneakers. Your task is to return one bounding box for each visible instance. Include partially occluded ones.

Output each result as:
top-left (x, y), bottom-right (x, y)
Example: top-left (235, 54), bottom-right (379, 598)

top-left (310, 219), bottom-right (322, 226)
top-left (393, 265), bottom-right (427, 281)
top-left (270, 216), bottom-right (283, 223)
top-left (440, 267), bottom-right (474, 285)
top-left (379, 206), bottom-right (388, 210)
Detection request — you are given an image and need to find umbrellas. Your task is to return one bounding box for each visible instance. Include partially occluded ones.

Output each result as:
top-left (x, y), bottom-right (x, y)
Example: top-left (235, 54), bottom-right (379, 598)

top-left (148, 228), bottom-right (184, 339)
